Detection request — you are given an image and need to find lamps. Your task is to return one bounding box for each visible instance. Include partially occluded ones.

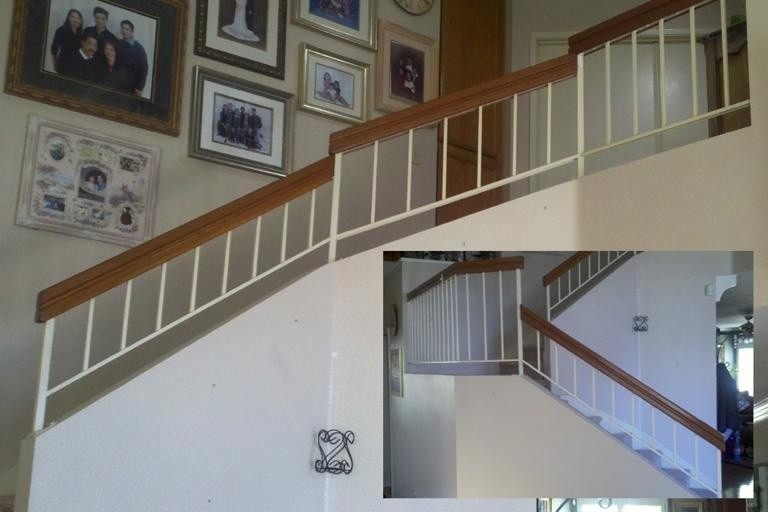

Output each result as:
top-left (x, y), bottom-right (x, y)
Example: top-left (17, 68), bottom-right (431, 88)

top-left (734, 313), bottom-right (754, 346)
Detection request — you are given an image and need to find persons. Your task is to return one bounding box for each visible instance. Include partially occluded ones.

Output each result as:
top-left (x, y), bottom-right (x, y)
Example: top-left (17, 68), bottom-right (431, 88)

top-left (85, 175), bottom-right (97, 192)
top-left (97, 175), bottom-right (107, 191)
top-left (316, 72), bottom-right (350, 106)
top-left (51, 6), bottom-right (148, 96)
top-left (399, 57), bottom-right (419, 98)
top-left (217, 102), bottom-right (262, 149)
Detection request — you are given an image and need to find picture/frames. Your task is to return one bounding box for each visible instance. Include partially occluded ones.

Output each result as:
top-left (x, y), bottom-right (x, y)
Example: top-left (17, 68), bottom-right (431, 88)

top-left (372, 15), bottom-right (439, 130)
top-left (3, 0), bottom-right (192, 140)
top-left (185, 62), bottom-right (298, 181)
top-left (289, 0), bottom-right (380, 54)
top-left (385, 347), bottom-right (403, 400)
top-left (190, 0), bottom-right (290, 83)
top-left (295, 40), bottom-right (372, 130)
top-left (12, 112), bottom-right (165, 251)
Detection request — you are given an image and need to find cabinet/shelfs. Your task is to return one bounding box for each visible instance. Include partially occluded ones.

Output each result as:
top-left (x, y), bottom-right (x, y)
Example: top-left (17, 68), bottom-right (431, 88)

top-left (695, 18), bottom-right (751, 138)
